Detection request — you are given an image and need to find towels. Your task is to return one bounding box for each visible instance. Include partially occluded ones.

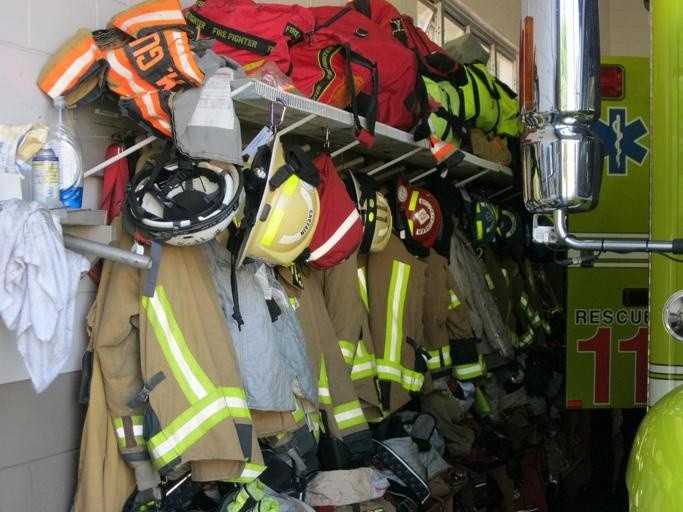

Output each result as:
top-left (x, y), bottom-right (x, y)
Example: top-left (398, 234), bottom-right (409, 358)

top-left (1, 200), bottom-right (91, 396)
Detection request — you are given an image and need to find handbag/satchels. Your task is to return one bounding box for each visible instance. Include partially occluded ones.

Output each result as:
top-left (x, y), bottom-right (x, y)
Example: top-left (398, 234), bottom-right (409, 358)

top-left (428, 111), bottom-right (462, 148)
top-left (290, 6), bottom-right (418, 148)
top-left (470, 125), bottom-right (514, 169)
top-left (421, 64), bottom-right (499, 134)
top-left (492, 75), bottom-right (523, 138)
top-left (183, 1), bottom-right (316, 78)
top-left (346, 0), bottom-right (468, 86)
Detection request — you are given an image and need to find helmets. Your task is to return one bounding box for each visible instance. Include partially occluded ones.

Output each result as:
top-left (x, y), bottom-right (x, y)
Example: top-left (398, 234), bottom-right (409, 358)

top-left (397, 179), bottom-right (442, 258)
top-left (349, 170), bottom-right (393, 252)
top-left (122, 156), bottom-right (244, 247)
top-left (226, 134), bottom-right (320, 270)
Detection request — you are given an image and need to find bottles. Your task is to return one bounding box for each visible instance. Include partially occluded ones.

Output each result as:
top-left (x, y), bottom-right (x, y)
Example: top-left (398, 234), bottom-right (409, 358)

top-left (33, 144), bottom-right (60, 210)
top-left (0, 152), bottom-right (32, 209)
top-left (44, 95), bottom-right (84, 210)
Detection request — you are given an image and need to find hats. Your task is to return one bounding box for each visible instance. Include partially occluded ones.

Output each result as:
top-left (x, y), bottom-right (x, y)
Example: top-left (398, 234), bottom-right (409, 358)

top-left (424, 175), bottom-right (460, 265)
top-left (305, 154), bottom-right (363, 270)
